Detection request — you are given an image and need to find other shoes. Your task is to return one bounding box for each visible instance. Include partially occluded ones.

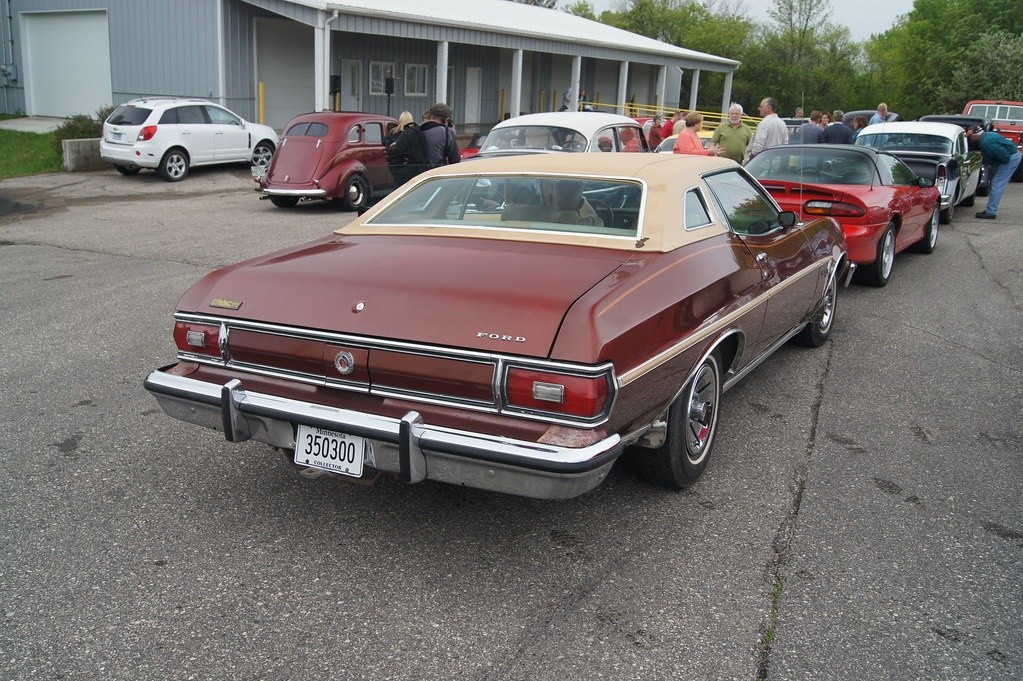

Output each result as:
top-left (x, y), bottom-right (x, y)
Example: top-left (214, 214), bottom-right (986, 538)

top-left (975, 211), bottom-right (996, 219)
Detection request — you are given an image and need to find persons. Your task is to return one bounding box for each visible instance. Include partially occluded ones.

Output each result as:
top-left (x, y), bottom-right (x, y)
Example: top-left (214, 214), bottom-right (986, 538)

top-left (800, 110), bottom-right (822, 144)
top-left (817, 112), bottom-right (831, 132)
top-left (560, 87), bottom-right (592, 105)
top-left (851, 116), bottom-right (868, 146)
top-left (674, 112), bottom-right (726, 157)
top-left (649, 113), bottom-right (663, 152)
top-left (598, 137), bottom-right (612, 152)
top-left (382, 103), bottom-right (461, 190)
top-left (964, 124), bottom-right (1021, 219)
top-left (868, 103), bottom-right (889, 147)
top-left (551, 105), bottom-right (575, 148)
top-left (619, 127), bottom-right (640, 152)
top-left (659, 111), bottom-right (689, 140)
top-left (711, 103), bottom-right (752, 165)
top-left (548, 180), bottom-right (605, 228)
top-left (744, 97), bottom-right (789, 162)
top-left (791, 107), bottom-right (810, 133)
top-left (819, 110), bottom-right (853, 144)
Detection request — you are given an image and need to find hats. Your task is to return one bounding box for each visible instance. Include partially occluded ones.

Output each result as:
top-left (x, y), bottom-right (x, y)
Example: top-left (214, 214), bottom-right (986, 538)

top-left (653, 114), bottom-right (663, 121)
top-left (430, 103), bottom-right (457, 125)
top-left (964, 124), bottom-right (983, 137)
top-left (831, 110), bottom-right (844, 118)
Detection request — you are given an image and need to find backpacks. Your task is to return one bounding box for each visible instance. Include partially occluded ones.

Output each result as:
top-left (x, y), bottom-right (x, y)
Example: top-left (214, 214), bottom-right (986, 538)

top-left (382, 121), bottom-right (445, 183)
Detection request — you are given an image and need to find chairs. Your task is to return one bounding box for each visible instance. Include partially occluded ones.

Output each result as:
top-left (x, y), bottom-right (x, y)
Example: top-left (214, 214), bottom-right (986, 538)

top-left (501, 205), bottom-right (559, 222)
top-left (843, 172), bottom-right (867, 183)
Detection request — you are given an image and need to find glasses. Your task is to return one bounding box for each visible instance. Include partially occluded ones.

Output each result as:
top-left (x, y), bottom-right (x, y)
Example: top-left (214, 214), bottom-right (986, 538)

top-left (658, 118), bottom-right (662, 121)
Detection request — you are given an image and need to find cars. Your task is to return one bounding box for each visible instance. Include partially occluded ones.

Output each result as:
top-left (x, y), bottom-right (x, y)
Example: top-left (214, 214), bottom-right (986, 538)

top-left (741, 144), bottom-right (943, 287)
top-left (100, 96), bottom-right (282, 182)
top-left (460, 101), bottom-right (1023, 226)
top-left (144, 153), bottom-right (855, 502)
top-left (255, 111), bottom-right (401, 211)
